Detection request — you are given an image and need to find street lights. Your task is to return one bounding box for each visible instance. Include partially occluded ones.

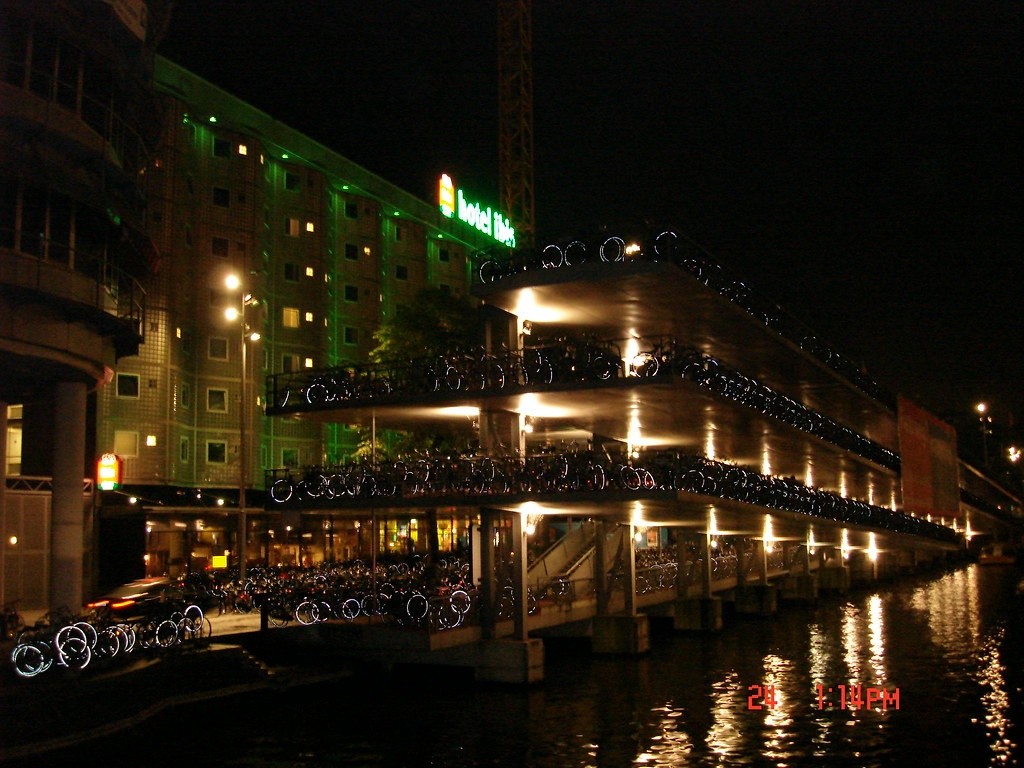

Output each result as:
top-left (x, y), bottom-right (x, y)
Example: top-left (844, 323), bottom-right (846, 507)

top-left (218, 266), bottom-right (261, 577)
top-left (976, 402), bottom-right (992, 472)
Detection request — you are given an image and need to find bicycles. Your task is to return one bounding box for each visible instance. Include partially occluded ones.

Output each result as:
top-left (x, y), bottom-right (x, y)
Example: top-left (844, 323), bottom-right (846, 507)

top-left (0, 594), bottom-right (212, 677)
top-left (639, 449), bottom-right (827, 517)
top-left (829, 493), bottom-right (958, 542)
top-left (164, 563), bottom-right (364, 625)
top-left (475, 220), bottom-right (676, 281)
top-left (614, 536), bottom-right (813, 591)
top-left (820, 415), bottom-right (901, 472)
top-left (959, 486), bottom-right (1010, 520)
top-left (358, 551), bottom-right (568, 630)
top-left (682, 348), bottom-right (817, 435)
top-left (680, 247), bottom-right (789, 333)
top-left (265, 337), bottom-right (669, 403)
top-left (796, 329), bottom-right (894, 407)
top-left (269, 441), bottom-right (639, 504)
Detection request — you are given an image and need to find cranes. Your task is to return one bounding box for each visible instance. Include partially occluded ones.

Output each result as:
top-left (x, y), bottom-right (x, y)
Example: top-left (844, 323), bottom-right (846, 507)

top-left (495, 0), bottom-right (534, 251)
